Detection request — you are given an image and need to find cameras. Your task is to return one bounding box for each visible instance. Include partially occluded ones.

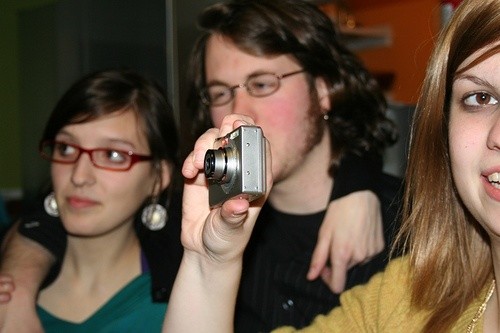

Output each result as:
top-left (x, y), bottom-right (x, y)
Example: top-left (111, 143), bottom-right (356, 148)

top-left (203, 125), bottom-right (266, 209)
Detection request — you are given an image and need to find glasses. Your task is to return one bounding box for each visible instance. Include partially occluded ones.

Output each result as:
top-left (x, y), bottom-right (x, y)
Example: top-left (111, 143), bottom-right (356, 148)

top-left (198, 68), bottom-right (306, 107)
top-left (38, 137), bottom-right (155, 171)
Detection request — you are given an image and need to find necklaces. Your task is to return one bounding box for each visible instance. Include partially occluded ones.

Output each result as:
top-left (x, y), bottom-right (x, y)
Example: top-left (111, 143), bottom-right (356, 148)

top-left (465, 279), bottom-right (496, 333)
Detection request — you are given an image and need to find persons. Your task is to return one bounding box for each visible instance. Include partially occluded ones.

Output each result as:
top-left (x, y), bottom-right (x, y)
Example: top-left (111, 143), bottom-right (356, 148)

top-left (159, 0), bottom-right (500, 333)
top-left (0, 0), bottom-right (412, 333)
